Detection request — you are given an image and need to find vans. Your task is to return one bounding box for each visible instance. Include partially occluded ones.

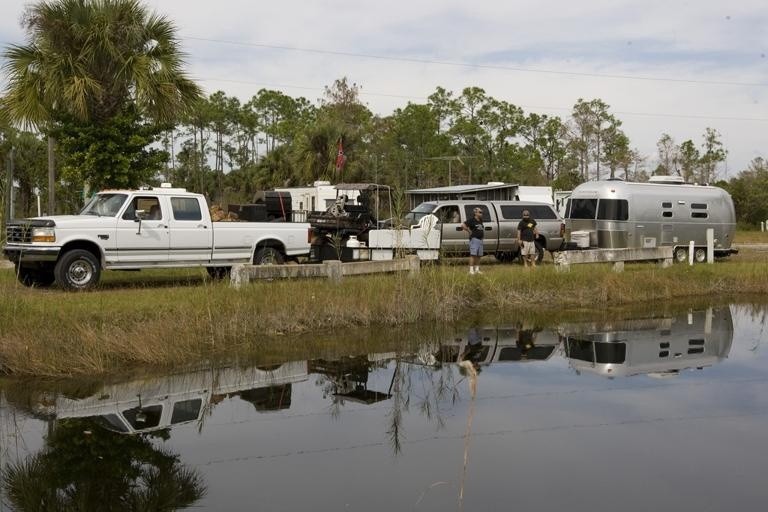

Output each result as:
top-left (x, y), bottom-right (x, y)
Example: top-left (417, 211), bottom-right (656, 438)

top-left (380, 200), bottom-right (566, 265)
top-left (406, 323), bottom-right (563, 371)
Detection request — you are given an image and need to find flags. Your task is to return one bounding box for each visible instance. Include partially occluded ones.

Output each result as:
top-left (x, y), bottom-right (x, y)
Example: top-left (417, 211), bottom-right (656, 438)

top-left (335, 141), bottom-right (345, 170)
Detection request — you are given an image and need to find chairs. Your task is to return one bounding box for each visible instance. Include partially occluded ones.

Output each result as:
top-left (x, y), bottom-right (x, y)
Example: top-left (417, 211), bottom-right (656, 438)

top-left (410, 213), bottom-right (441, 249)
top-left (449, 211), bottom-right (459, 221)
top-left (147, 207), bottom-right (161, 220)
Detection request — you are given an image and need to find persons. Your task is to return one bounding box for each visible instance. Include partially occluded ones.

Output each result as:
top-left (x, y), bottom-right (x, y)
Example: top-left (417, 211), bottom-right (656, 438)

top-left (460, 326), bottom-right (484, 375)
top-left (461, 207), bottom-right (486, 275)
top-left (517, 209), bottom-right (540, 268)
top-left (515, 319), bottom-right (542, 363)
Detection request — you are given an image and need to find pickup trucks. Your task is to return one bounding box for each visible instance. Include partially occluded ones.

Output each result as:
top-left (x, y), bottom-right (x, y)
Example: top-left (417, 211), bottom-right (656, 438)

top-left (1, 183), bottom-right (313, 292)
top-left (6, 360), bottom-right (309, 445)
